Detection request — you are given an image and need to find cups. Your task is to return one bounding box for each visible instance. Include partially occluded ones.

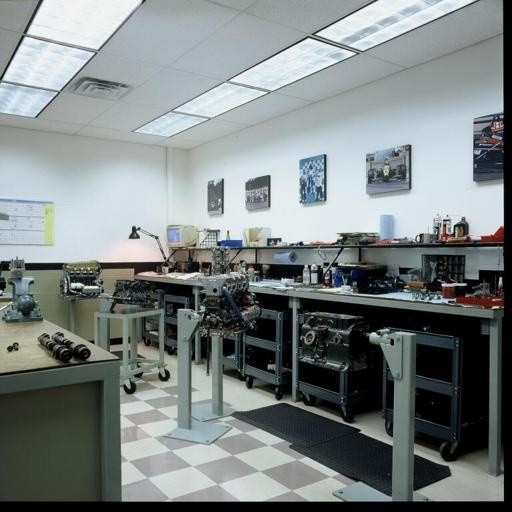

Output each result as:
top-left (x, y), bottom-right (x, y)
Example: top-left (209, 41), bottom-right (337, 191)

top-left (162, 267), bottom-right (169, 273)
top-left (380, 214), bottom-right (394, 242)
top-left (415, 233), bottom-right (431, 243)
top-left (201, 262), bottom-right (211, 277)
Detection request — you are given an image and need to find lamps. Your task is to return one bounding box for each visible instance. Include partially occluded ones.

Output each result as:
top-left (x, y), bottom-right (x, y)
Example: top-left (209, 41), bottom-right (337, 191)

top-left (128, 226), bottom-right (172, 274)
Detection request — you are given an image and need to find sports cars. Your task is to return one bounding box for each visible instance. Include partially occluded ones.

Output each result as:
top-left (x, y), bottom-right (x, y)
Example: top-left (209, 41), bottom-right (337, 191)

top-left (247, 190), bottom-right (268, 203)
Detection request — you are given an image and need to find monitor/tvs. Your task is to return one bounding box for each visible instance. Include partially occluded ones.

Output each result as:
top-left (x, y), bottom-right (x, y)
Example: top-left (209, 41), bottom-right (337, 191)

top-left (165, 224), bottom-right (199, 249)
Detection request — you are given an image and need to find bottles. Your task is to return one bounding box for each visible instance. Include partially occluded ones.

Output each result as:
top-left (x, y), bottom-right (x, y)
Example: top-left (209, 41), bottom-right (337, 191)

top-left (225, 230), bottom-right (231, 240)
top-left (295, 260), bottom-right (339, 288)
top-left (431, 213), bottom-right (470, 244)
top-left (212, 245), bottom-right (231, 275)
top-left (234, 259), bottom-right (269, 282)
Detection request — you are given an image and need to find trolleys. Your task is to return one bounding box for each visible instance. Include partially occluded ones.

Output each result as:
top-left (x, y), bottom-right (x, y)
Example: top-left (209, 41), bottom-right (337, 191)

top-left (92, 291), bottom-right (291, 400)
top-left (297, 310), bottom-right (378, 423)
top-left (381, 324), bottom-right (484, 461)
